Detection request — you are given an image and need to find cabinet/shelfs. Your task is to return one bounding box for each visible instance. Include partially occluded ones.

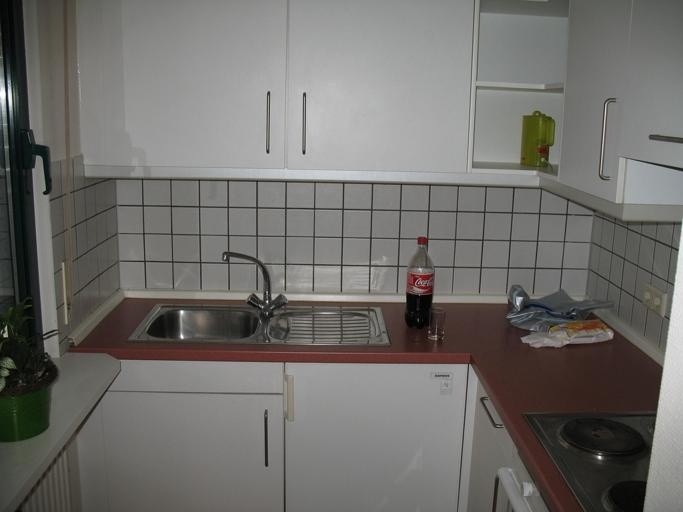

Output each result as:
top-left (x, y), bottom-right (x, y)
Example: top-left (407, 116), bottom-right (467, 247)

top-left (284, 362), bottom-right (467, 510)
top-left (71, 360), bottom-right (283, 510)
top-left (74, 1), bottom-right (475, 184)
top-left (558, 1), bottom-right (633, 208)
top-left (618, 0), bottom-right (683, 170)
top-left (472, 0), bottom-right (568, 178)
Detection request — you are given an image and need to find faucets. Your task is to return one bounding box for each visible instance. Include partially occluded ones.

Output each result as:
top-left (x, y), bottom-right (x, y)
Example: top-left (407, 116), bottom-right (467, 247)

top-left (222, 252), bottom-right (271, 303)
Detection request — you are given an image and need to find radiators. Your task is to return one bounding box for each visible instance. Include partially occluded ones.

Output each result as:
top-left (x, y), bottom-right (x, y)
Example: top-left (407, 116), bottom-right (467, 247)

top-left (16, 449), bottom-right (70, 512)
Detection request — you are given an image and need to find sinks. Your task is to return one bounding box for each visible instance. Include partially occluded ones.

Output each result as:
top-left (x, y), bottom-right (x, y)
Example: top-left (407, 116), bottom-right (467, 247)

top-left (128, 303), bottom-right (260, 346)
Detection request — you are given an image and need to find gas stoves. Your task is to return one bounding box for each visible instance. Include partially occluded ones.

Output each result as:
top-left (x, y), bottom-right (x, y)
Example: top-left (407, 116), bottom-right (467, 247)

top-left (520, 413), bottom-right (659, 511)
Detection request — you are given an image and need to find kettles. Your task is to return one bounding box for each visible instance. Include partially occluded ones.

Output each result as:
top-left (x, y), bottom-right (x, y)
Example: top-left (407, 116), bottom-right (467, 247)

top-left (521, 111), bottom-right (555, 167)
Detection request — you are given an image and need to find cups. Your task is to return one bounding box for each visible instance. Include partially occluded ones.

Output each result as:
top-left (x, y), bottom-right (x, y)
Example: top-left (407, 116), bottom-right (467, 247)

top-left (424, 307), bottom-right (446, 343)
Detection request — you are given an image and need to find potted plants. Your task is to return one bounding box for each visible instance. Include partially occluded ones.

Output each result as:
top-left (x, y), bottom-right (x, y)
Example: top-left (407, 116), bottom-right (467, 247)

top-left (0, 299), bottom-right (59, 440)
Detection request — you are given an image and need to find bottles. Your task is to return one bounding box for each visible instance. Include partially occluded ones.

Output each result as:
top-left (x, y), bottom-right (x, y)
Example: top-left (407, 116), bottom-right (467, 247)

top-left (405, 236), bottom-right (435, 328)
